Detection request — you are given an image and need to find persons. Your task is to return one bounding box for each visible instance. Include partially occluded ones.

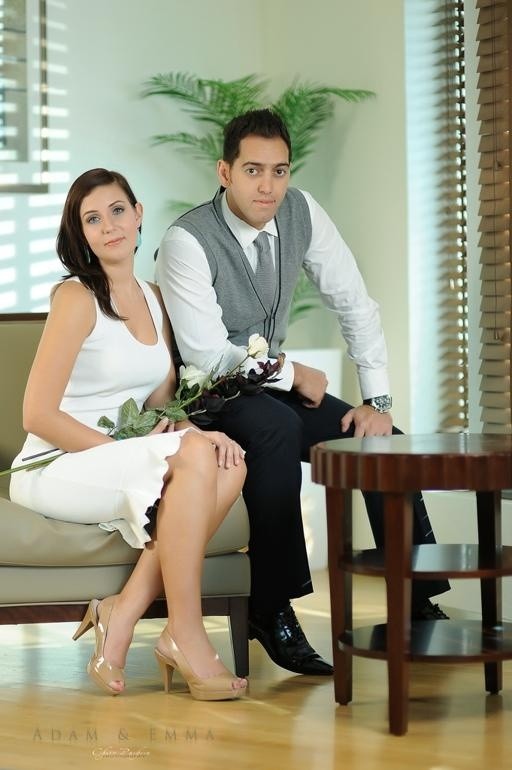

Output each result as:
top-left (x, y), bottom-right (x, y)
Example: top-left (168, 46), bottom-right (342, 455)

top-left (9, 168), bottom-right (248, 701)
top-left (155, 109), bottom-right (452, 675)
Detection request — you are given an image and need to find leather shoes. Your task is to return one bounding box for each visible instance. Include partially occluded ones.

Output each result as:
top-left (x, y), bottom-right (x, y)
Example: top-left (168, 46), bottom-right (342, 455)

top-left (411, 602), bottom-right (450, 621)
top-left (247, 605), bottom-right (334, 675)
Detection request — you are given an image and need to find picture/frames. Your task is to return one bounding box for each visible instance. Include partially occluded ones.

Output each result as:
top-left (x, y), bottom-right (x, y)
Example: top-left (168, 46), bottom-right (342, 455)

top-left (0, 1), bottom-right (51, 194)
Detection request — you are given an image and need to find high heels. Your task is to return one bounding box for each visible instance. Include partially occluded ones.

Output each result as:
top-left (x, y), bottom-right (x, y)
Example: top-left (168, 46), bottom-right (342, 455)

top-left (153, 625), bottom-right (248, 700)
top-left (72, 595), bottom-right (129, 695)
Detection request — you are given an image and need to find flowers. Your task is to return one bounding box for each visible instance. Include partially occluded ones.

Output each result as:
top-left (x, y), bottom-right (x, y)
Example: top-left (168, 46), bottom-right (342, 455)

top-left (2, 330), bottom-right (286, 490)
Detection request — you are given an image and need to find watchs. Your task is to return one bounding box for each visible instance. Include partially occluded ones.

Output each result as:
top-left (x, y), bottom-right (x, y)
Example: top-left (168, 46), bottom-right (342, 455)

top-left (363, 394), bottom-right (393, 415)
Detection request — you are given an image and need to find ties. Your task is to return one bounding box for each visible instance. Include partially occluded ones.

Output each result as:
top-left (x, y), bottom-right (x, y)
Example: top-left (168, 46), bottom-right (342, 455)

top-left (253, 232), bottom-right (277, 311)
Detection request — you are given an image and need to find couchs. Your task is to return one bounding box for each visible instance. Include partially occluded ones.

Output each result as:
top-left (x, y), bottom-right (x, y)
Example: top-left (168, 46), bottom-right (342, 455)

top-left (2, 311), bottom-right (251, 682)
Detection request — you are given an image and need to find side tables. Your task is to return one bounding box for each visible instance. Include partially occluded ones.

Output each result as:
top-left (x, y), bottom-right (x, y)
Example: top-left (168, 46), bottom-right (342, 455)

top-left (309, 432), bottom-right (512, 733)
